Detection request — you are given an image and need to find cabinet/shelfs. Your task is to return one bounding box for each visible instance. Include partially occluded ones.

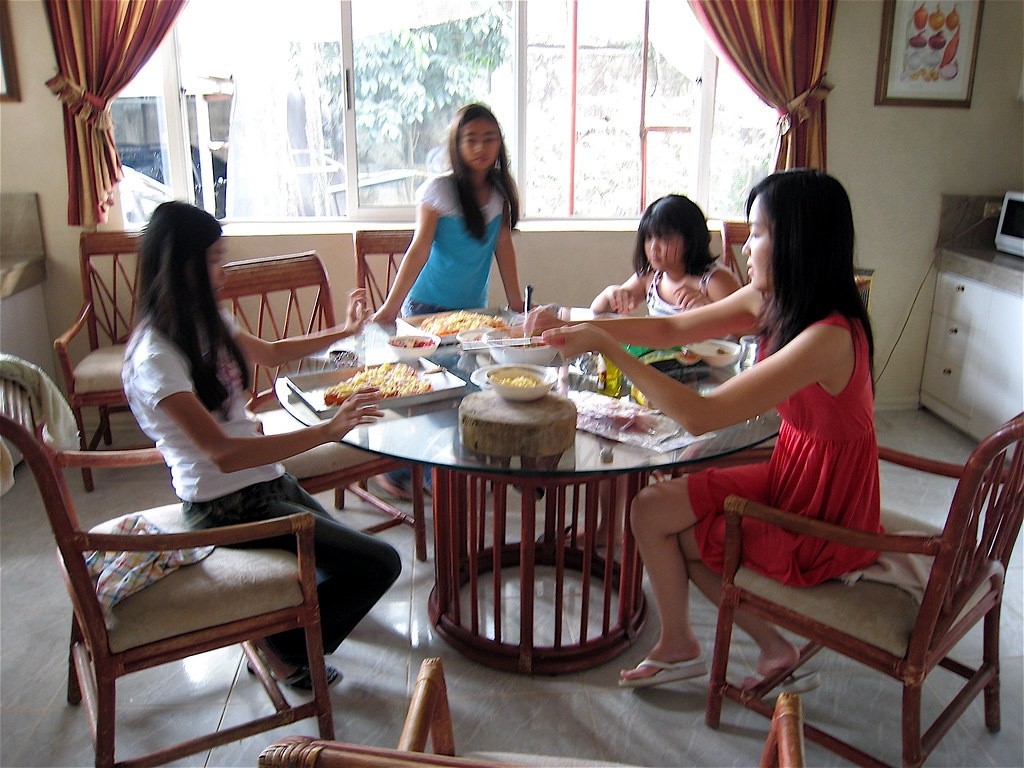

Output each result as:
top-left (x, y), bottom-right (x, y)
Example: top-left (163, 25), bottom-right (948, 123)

top-left (920, 269), bottom-right (1023, 463)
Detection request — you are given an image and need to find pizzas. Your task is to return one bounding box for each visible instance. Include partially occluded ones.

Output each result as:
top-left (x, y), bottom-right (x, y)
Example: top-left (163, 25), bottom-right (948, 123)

top-left (324, 362), bottom-right (433, 405)
top-left (420, 309), bottom-right (507, 335)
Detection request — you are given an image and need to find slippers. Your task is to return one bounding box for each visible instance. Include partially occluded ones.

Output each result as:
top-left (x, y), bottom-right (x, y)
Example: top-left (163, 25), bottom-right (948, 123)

top-left (617, 658), bottom-right (707, 687)
top-left (741, 637), bottom-right (823, 705)
top-left (247, 638), bottom-right (344, 697)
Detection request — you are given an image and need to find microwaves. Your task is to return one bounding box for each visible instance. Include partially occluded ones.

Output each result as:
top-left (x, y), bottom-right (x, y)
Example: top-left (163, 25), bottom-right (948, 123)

top-left (996, 191), bottom-right (1024, 257)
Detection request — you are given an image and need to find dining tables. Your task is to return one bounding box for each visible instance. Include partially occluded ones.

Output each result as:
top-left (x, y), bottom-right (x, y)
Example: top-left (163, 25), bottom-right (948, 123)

top-left (273, 307), bottom-right (777, 677)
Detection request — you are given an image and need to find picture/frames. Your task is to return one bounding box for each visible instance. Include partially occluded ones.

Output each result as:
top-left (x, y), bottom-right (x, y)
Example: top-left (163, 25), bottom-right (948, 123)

top-left (874, 0), bottom-right (985, 111)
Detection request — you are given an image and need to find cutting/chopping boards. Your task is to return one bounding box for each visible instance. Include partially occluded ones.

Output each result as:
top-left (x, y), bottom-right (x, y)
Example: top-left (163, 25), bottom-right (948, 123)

top-left (459, 392), bottom-right (577, 456)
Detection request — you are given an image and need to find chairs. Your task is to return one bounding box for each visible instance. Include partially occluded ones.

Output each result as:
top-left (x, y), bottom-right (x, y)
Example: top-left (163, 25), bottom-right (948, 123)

top-left (353, 229), bottom-right (415, 501)
top-left (52, 231), bottom-right (143, 492)
top-left (216, 249), bottom-right (426, 562)
top-left (0, 375), bottom-right (334, 768)
top-left (703, 413), bottom-right (1024, 768)
top-left (256, 656), bottom-right (807, 768)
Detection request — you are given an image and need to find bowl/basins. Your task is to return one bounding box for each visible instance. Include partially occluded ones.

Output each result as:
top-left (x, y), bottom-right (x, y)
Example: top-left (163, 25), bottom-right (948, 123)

top-left (689, 339), bottom-right (740, 368)
top-left (484, 330), bottom-right (558, 366)
top-left (456, 329), bottom-right (488, 354)
top-left (386, 334), bottom-right (441, 356)
top-left (485, 364), bottom-right (559, 401)
top-left (470, 363), bottom-right (499, 392)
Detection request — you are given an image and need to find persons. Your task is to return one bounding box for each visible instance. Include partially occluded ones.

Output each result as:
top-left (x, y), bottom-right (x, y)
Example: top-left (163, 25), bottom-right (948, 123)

top-left (371, 104), bottom-right (538, 502)
top-left (564, 194), bottom-right (739, 546)
top-left (525, 170), bottom-right (888, 693)
top-left (122, 199), bottom-right (403, 693)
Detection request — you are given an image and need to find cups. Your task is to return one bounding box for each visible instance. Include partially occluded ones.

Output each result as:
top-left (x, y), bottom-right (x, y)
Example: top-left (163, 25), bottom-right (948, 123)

top-left (740, 335), bottom-right (758, 372)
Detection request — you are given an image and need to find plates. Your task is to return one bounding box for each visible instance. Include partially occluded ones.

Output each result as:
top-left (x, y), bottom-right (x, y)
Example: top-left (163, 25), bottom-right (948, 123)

top-left (475, 354), bottom-right (499, 367)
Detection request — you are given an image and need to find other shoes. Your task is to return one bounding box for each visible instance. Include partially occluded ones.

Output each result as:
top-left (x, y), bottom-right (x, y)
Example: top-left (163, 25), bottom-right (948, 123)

top-left (375, 468), bottom-right (413, 500)
